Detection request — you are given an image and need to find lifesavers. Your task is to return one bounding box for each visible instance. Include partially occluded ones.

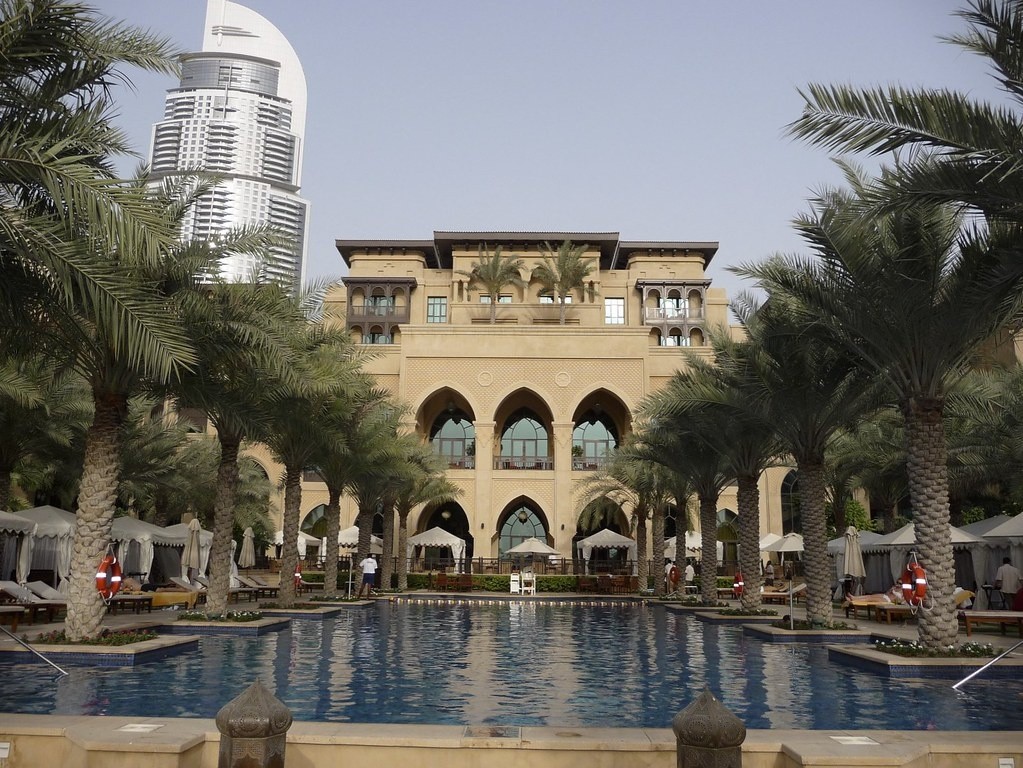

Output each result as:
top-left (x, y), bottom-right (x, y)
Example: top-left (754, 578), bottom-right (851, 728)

top-left (733, 572), bottom-right (744, 593)
top-left (901, 561), bottom-right (926, 607)
top-left (669, 567), bottom-right (679, 582)
top-left (294, 565), bottom-right (302, 587)
top-left (97, 554), bottom-right (122, 599)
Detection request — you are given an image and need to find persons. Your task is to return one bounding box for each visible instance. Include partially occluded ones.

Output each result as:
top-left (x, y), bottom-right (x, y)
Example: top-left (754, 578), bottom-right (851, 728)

top-left (684, 562), bottom-right (695, 594)
top-left (785, 565), bottom-right (792, 581)
top-left (665, 560), bottom-right (675, 594)
top-left (992, 556), bottom-right (1023, 612)
top-left (765, 561), bottom-right (774, 586)
top-left (357, 553), bottom-right (378, 602)
top-left (844, 577), bottom-right (904, 602)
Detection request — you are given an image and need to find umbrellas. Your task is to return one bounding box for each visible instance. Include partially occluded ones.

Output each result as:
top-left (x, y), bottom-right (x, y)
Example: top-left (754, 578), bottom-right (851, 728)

top-left (983, 511), bottom-right (1023, 547)
top-left (182, 519), bottom-right (201, 583)
top-left (825, 529), bottom-right (892, 590)
top-left (238, 527), bottom-right (256, 579)
top-left (872, 520), bottom-right (988, 555)
top-left (503, 536), bottom-right (564, 574)
top-left (843, 526), bottom-right (866, 586)
top-left (957, 514), bottom-right (1014, 593)
top-left (760, 533), bottom-right (805, 577)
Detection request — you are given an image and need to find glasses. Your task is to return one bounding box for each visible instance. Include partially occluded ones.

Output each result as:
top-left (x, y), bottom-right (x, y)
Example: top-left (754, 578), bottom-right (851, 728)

top-left (897, 581), bottom-right (902, 585)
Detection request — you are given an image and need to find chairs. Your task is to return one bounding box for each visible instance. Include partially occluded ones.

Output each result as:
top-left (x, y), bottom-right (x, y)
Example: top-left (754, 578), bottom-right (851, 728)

top-left (611, 572), bottom-right (627, 594)
top-left (63, 575), bottom-right (154, 614)
top-left (508, 571), bottom-right (520, 595)
top-left (193, 576), bottom-right (259, 602)
top-left (716, 574), bottom-right (1023, 638)
top-left (23, 580), bottom-right (116, 617)
top-left (596, 574), bottom-right (611, 592)
top-left (576, 576), bottom-right (594, 593)
top-left (122, 589), bottom-right (201, 612)
top-left (520, 566), bottom-right (537, 595)
top-left (458, 573), bottom-right (472, 592)
top-left (169, 576), bottom-right (239, 604)
top-left (235, 575), bottom-right (302, 597)
top-left (299, 579), bottom-right (325, 593)
top-left (249, 574), bottom-right (280, 596)
top-left (0, 579), bottom-right (68, 624)
top-left (436, 572), bottom-right (448, 591)
top-left (0, 604), bottom-right (26, 633)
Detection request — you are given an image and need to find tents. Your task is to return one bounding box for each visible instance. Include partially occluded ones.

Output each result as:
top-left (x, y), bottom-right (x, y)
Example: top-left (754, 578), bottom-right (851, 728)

top-left (267, 529), bottom-right (322, 565)
top-left (575, 528), bottom-right (637, 575)
top-left (337, 525), bottom-right (383, 554)
top-left (405, 527), bottom-right (469, 573)
top-left (108, 514), bottom-right (188, 585)
top-left (662, 530), bottom-right (723, 567)
top-left (0, 510), bottom-right (38, 588)
top-left (12, 505), bottom-right (77, 589)
top-left (166, 523), bottom-right (242, 589)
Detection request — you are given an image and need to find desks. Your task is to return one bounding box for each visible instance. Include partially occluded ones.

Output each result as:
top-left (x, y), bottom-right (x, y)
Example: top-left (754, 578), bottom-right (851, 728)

top-left (432, 580), bottom-right (473, 592)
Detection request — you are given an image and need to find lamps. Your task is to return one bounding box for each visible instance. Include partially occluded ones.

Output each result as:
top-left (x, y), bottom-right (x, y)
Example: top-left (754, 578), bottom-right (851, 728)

top-left (441, 511), bottom-right (451, 519)
top-left (518, 505), bottom-right (528, 522)
